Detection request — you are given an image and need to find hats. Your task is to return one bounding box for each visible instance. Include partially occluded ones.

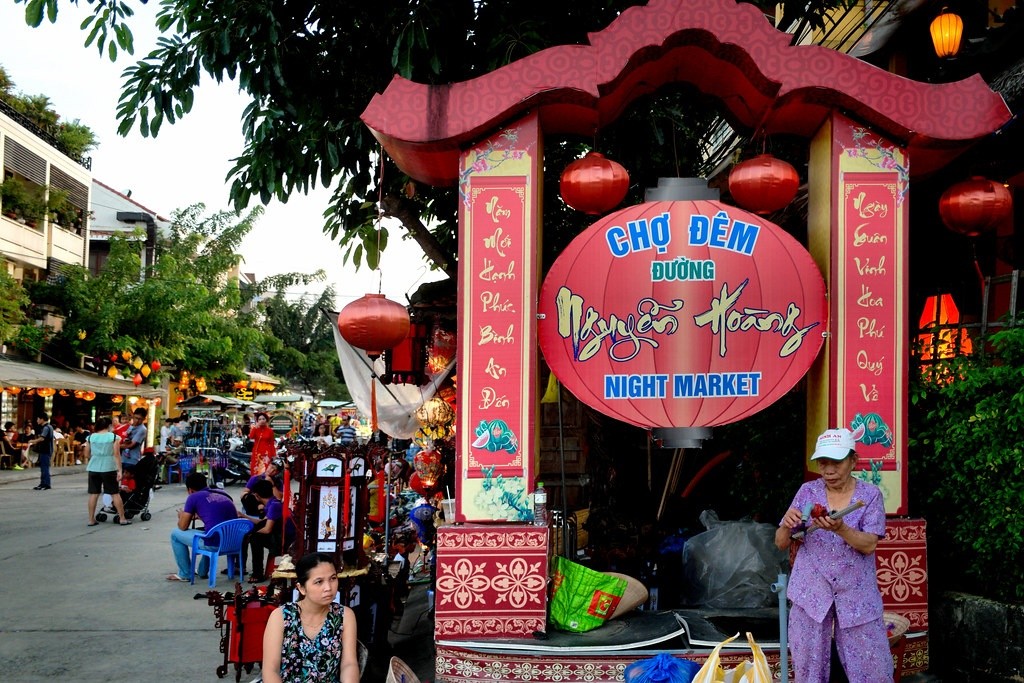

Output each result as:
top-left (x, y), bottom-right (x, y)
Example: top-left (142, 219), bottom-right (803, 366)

top-left (386, 657), bottom-right (422, 683)
top-left (811, 427), bottom-right (856, 460)
top-left (831, 612), bottom-right (910, 647)
top-left (271, 459), bottom-right (284, 472)
top-left (600, 572), bottom-right (648, 620)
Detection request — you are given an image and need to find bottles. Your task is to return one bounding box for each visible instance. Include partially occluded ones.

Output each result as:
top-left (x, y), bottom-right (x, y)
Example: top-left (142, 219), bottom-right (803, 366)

top-left (534, 482), bottom-right (548, 527)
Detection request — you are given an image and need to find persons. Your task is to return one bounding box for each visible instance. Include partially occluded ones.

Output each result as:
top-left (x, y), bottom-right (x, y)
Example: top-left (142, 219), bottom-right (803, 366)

top-left (155, 411), bottom-right (192, 484)
top-left (3, 420), bottom-right (90, 471)
top-left (254, 552), bottom-right (359, 683)
top-left (84, 408), bottom-right (147, 526)
top-left (247, 412), bottom-right (277, 478)
top-left (775, 427), bottom-right (895, 683)
top-left (28, 413), bottom-right (53, 491)
top-left (303, 408), bottom-right (358, 449)
top-left (165, 458), bottom-right (295, 583)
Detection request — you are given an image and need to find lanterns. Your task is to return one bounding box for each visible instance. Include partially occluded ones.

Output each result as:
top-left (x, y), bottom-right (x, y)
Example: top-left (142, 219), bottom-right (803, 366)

top-left (277, 437), bottom-right (372, 574)
top-left (560, 132), bottom-right (630, 216)
top-left (337, 268), bottom-right (455, 432)
top-left (728, 135), bottom-right (799, 215)
top-left (913, 294), bottom-right (973, 385)
top-left (407, 394), bottom-right (453, 574)
top-left (939, 176), bottom-right (1012, 303)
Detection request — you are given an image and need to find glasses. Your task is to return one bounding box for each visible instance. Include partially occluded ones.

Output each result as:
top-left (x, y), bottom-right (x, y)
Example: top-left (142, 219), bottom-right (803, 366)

top-left (134, 416), bottom-right (144, 421)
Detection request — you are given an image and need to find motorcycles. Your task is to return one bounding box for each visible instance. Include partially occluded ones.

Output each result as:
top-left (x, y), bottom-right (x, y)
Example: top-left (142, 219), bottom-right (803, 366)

top-left (219, 450), bottom-right (284, 488)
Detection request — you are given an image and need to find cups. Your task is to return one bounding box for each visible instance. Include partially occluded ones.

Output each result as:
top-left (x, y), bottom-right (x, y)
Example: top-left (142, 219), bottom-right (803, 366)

top-left (442, 499), bottom-right (455, 524)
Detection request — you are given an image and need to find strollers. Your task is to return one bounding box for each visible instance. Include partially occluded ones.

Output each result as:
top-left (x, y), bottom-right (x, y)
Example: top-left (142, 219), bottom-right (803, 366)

top-left (95, 451), bottom-right (167, 525)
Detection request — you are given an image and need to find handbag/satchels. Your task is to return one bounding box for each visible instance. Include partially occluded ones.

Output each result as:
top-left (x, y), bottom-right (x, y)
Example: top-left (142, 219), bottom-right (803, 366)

top-left (624, 653), bottom-right (699, 683)
top-left (691, 632), bottom-right (775, 682)
top-left (549, 555), bottom-right (626, 632)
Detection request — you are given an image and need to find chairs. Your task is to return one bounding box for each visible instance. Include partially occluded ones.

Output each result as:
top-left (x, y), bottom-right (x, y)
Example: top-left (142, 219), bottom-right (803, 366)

top-left (0, 437), bottom-right (75, 472)
top-left (169, 460), bottom-right (188, 484)
top-left (191, 518), bottom-right (255, 587)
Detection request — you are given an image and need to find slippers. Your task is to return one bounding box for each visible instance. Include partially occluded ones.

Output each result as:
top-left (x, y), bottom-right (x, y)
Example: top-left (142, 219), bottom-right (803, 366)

top-left (220, 568), bottom-right (249, 576)
top-left (195, 569), bottom-right (209, 579)
top-left (88, 520), bottom-right (99, 526)
top-left (166, 575), bottom-right (191, 582)
top-left (247, 574), bottom-right (268, 583)
top-left (120, 520), bottom-right (133, 525)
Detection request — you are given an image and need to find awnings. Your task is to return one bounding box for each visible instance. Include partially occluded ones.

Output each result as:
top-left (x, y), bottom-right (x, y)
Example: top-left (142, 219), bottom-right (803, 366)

top-left (0, 360), bottom-right (168, 406)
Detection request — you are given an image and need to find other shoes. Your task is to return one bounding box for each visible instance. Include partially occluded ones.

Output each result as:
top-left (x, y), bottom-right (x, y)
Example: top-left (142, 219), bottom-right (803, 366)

top-left (13, 464), bottom-right (24, 470)
top-left (76, 459), bottom-right (81, 465)
top-left (34, 484), bottom-right (51, 490)
